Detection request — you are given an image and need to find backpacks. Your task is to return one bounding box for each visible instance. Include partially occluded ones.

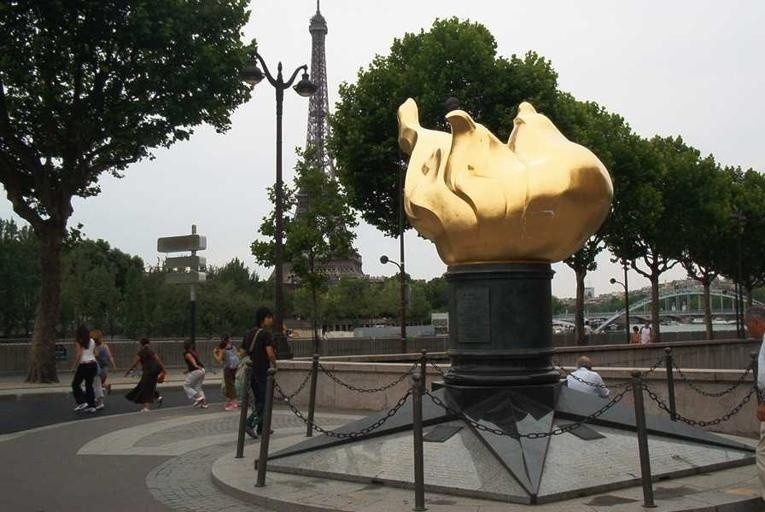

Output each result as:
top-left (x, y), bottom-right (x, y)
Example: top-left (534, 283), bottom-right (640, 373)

top-left (222, 345), bottom-right (240, 370)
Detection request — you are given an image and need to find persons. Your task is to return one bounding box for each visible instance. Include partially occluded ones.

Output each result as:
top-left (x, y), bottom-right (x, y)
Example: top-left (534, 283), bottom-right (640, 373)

top-left (639, 321), bottom-right (653, 344)
top-left (743, 305), bottom-right (765, 506)
top-left (630, 326), bottom-right (639, 343)
top-left (71, 327), bottom-right (97, 413)
top-left (90, 329), bottom-right (117, 393)
top-left (180, 338), bottom-right (207, 409)
top-left (567, 354), bottom-right (610, 400)
top-left (123, 338), bottom-right (167, 411)
top-left (213, 333), bottom-right (241, 411)
top-left (243, 307), bottom-right (279, 438)
top-left (584, 321), bottom-right (593, 335)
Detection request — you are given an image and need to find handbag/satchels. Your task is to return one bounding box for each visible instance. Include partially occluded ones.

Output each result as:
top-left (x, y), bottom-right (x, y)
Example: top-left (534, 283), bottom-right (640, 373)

top-left (99, 350), bottom-right (108, 368)
top-left (157, 370), bottom-right (166, 383)
top-left (235, 355), bottom-right (254, 384)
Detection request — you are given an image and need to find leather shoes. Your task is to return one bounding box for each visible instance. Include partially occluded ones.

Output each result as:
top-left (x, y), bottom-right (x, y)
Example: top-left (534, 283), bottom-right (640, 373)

top-left (245, 425), bottom-right (258, 439)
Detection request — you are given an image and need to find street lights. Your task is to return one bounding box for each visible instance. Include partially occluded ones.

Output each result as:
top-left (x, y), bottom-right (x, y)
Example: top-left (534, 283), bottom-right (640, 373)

top-left (240, 49), bottom-right (319, 362)
top-left (722, 289), bottom-right (742, 339)
top-left (611, 278), bottom-right (631, 345)
top-left (380, 254), bottom-right (408, 353)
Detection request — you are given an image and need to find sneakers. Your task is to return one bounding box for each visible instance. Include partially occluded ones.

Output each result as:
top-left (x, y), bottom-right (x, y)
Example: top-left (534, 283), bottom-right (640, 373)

top-left (73, 384), bottom-right (241, 413)
